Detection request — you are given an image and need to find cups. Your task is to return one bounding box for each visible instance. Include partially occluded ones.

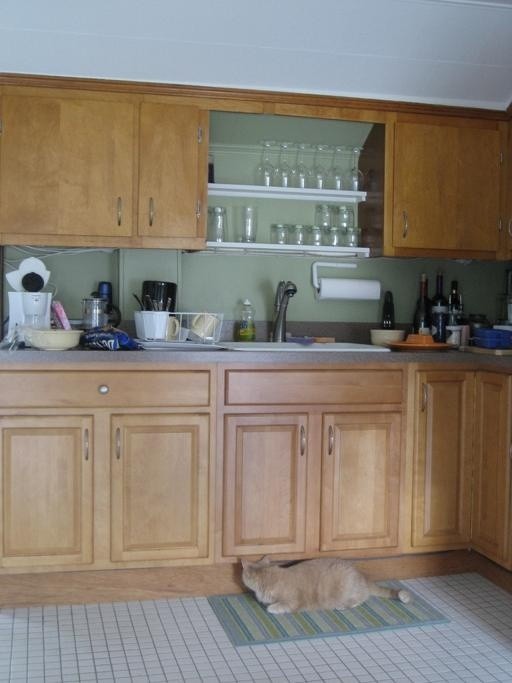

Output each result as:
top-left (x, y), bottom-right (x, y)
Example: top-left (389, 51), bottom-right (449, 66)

top-left (333, 205), bottom-right (355, 227)
top-left (345, 142), bottom-right (365, 190)
top-left (314, 203), bottom-right (337, 226)
top-left (232, 203), bottom-right (259, 242)
top-left (290, 222), bottom-right (310, 244)
top-left (271, 222), bottom-right (290, 244)
top-left (290, 142), bottom-right (311, 188)
top-left (274, 140), bottom-right (294, 186)
top-left (133, 310), bottom-right (170, 340)
top-left (326, 143), bottom-right (347, 189)
top-left (306, 223), bottom-right (325, 244)
top-left (206, 206), bottom-right (229, 242)
top-left (310, 142), bottom-right (329, 188)
top-left (166, 316), bottom-right (180, 340)
top-left (254, 139), bottom-right (277, 185)
top-left (328, 225), bottom-right (346, 246)
top-left (191, 308), bottom-right (220, 341)
top-left (346, 227), bottom-right (360, 246)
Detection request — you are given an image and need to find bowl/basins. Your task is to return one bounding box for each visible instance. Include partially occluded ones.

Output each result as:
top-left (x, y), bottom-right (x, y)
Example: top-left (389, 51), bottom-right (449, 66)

top-left (369, 329), bottom-right (404, 347)
top-left (28, 330), bottom-right (84, 350)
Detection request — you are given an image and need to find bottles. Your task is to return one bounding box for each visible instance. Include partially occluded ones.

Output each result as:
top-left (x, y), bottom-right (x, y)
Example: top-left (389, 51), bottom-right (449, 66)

top-left (449, 280), bottom-right (460, 314)
top-left (414, 273), bottom-right (433, 333)
top-left (434, 270), bottom-right (448, 342)
top-left (382, 290), bottom-right (394, 329)
top-left (238, 300), bottom-right (255, 340)
top-left (98, 282), bottom-right (111, 324)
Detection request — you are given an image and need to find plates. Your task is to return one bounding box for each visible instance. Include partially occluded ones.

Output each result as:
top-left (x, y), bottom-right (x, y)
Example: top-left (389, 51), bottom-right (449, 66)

top-left (384, 333), bottom-right (458, 350)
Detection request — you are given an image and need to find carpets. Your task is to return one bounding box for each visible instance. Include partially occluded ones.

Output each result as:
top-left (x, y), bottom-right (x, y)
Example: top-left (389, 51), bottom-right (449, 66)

top-left (204, 578), bottom-right (452, 649)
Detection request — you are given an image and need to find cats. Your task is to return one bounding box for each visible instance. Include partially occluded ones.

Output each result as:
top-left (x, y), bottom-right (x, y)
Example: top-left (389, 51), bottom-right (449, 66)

top-left (240, 554), bottom-right (413, 617)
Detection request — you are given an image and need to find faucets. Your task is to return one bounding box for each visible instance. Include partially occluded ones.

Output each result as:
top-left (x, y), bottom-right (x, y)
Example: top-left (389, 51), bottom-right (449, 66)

top-left (272, 281), bottom-right (298, 342)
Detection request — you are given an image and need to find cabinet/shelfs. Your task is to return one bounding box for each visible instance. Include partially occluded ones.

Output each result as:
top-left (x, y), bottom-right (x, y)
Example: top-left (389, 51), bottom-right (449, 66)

top-left (0, 368), bottom-right (216, 574)
top-left (410, 368), bottom-right (470, 549)
top-left (497, 118), bottom-right (511, 260)
top-left (0, 83), bottom-right (209, 249)
top-left (470, 374), bottom-right (512, 577)
top-left (215, 362), bottom-right (406, 564)
top-left (358, 114), bottom-right (503, 265)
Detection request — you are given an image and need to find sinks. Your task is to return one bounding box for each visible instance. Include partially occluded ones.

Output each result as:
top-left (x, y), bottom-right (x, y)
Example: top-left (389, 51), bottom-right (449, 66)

top-left (219, 341), bottom-right (392, 353)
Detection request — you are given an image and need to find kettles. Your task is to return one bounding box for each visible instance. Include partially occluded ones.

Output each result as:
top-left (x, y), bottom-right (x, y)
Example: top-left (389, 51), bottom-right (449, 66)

top-left (82, 297), bottom-right (121, 331)
